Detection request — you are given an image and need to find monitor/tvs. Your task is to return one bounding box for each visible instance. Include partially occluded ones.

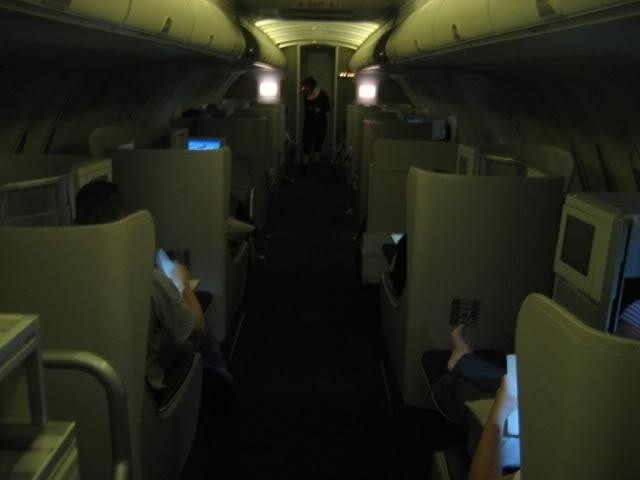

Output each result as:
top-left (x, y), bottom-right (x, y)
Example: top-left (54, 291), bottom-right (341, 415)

top-left (455, 144), bottom-right (528, 176)
top-left (552, 189), bottom-right (639, 304)
top-left (184, 135), bottom-right (228, 150)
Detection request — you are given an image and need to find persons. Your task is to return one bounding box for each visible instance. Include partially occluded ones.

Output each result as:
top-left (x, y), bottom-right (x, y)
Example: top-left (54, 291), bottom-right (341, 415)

top-left (285, 105), bottom-right (296, 158)
top-left (381, 170), bottom-right (445, 277)
top-left (439, 322), bottom-right (522, 479)
top-left (301, 77), bottom-right (330, 165)
top-left (75, 181), bottom-right (205, 400)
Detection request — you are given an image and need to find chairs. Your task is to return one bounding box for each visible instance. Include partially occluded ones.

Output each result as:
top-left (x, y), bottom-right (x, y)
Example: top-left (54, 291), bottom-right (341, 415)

top-left (345, 102), bottom-right (639, 480)
top-left (0, 99), bottom-right (286, 480)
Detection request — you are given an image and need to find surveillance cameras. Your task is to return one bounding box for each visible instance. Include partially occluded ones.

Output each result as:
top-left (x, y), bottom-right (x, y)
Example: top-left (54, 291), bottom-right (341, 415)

top-left (248, 48), bottom-right (254, 56)
